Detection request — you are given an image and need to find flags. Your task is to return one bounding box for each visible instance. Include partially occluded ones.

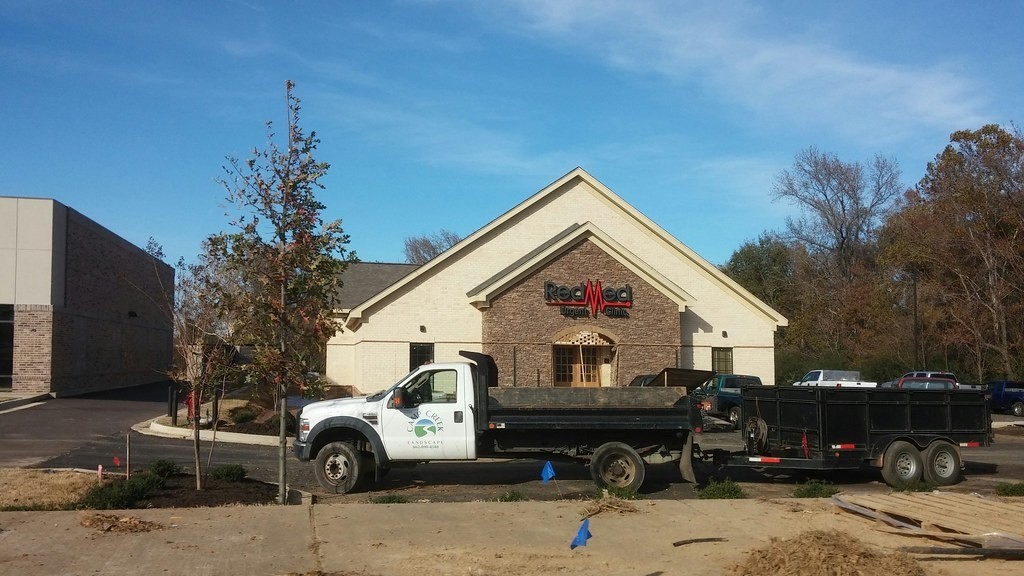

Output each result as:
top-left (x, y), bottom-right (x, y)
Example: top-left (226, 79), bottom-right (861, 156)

top-left (570, 519), bottom-right (592, 551)
top-left (540, 461), bottom-right (555, 485)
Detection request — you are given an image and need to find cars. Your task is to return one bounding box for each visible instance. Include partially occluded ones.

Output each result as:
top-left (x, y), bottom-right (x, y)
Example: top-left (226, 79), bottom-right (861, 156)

top-left (628, 375), bottom-right (704, 434)
top-left (890, 378), bottom-right (959, 390)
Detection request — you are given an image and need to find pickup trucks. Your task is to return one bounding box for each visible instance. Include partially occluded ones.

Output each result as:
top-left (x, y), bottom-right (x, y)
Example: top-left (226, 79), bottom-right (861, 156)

top-left (793, 370), bottom-right (877, 389)
top-left (693, 375), bottom-right (763, 430)
top-left (980, 381), bottom-right (1023, 417)
top-left (882, 371), bottom-right (988, 397)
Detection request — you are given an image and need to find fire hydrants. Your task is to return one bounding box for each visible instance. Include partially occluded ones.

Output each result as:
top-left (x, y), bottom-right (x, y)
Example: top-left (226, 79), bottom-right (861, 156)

top-left (184, 389), bottom-right (205, 424)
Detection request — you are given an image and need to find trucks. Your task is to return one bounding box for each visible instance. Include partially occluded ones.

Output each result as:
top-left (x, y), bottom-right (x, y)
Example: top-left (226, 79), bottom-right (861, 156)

top-left (290, 349), bottom-right (704, 502)
